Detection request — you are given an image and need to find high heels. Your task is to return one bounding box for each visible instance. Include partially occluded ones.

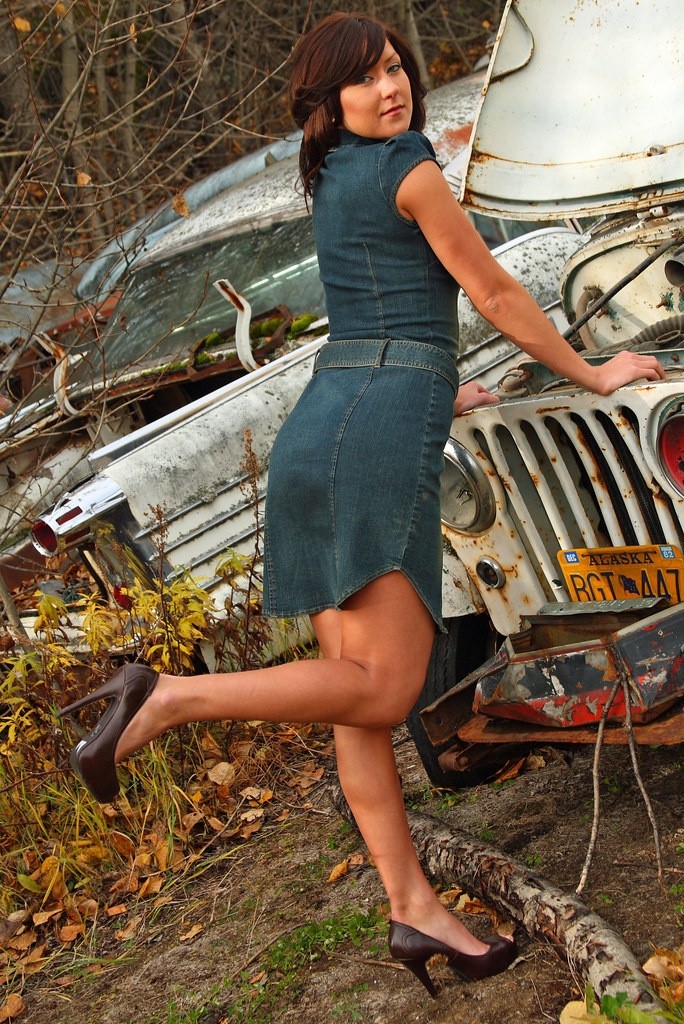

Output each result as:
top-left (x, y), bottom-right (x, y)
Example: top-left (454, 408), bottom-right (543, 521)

top-left (56, 662), bottom-right (160, 805)
top-left (388, 916), bottom-right (516, 996)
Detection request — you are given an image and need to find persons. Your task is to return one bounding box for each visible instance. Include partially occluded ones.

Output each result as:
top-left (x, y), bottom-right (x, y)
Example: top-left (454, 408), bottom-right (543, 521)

top-left (56, 13), bottom-right (666, 998)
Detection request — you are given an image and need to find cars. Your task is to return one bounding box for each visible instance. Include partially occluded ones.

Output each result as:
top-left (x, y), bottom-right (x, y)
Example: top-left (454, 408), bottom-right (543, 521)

top-left (1, 65), bottom-right (684, 788)
